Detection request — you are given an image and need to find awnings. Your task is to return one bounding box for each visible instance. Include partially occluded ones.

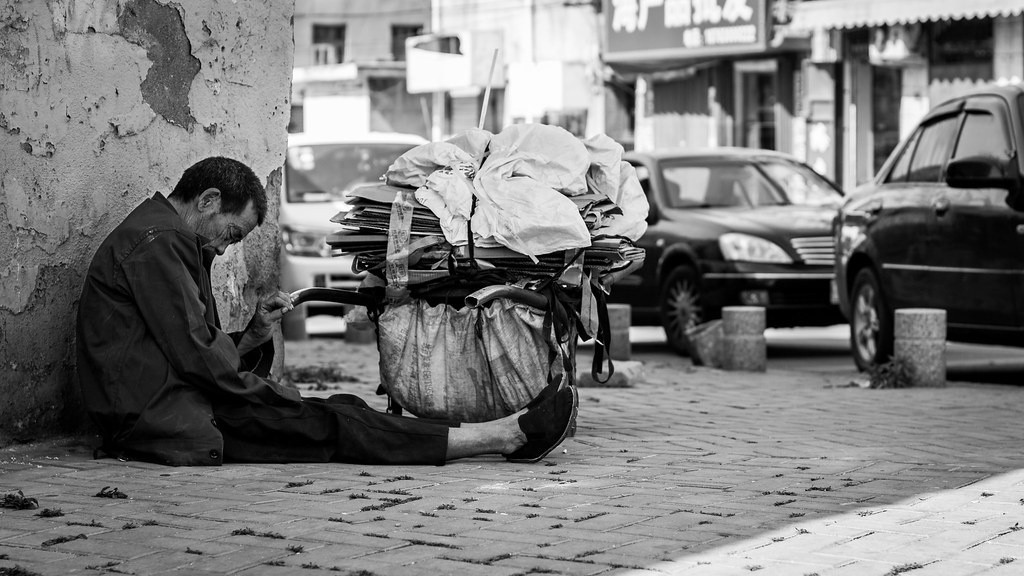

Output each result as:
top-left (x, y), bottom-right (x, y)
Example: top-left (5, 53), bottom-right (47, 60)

top-left (774, 0), bottom-right (1024, 53)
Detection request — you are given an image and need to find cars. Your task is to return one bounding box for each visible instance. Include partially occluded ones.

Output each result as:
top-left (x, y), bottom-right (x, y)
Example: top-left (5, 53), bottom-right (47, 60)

top-left (836, 85), bottom-right (1024, 372)
top-left (280, 131), bottom-right (434, 315)
top-left (603, 146), bottom-right (850, 357)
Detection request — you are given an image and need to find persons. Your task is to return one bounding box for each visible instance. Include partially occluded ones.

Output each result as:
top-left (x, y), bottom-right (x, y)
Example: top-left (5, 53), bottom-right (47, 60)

top-left (75, 157), bottom-right (578, 466)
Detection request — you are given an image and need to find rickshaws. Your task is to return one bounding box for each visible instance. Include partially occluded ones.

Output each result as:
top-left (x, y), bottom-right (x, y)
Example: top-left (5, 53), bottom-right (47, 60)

top-left (282, 256), bottom-right (645, 439)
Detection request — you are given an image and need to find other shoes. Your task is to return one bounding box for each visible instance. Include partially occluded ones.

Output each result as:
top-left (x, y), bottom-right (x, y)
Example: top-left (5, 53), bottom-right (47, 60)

top-left (501, 385), bottom-right (578, 463)
top-left (519, 372), bottom-right (566, 413)
